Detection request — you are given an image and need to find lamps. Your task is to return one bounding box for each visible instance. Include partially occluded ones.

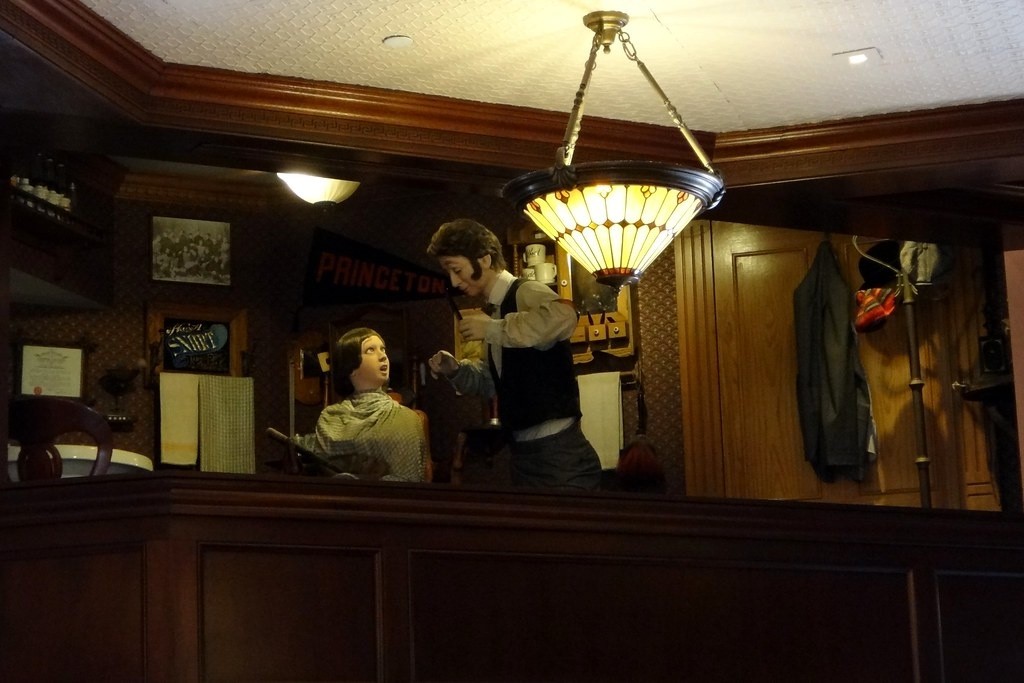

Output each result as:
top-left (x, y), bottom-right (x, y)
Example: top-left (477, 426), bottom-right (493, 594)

top-left (277, 172), bottom-right (361, 213)
top-left (501, 10), bottom-right (726, 297)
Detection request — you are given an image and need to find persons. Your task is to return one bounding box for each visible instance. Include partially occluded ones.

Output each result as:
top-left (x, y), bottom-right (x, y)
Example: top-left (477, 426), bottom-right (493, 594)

top-left (152, 229), bottom-right (231, 284)
top-left (288, 327), bottom-right (423, 482)
top-left (426, 219), bottom-right (603, 492)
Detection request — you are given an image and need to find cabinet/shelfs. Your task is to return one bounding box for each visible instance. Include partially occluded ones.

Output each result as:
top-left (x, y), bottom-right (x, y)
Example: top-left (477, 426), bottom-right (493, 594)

top-left (0, 470), bottom-right (1024, 683)
